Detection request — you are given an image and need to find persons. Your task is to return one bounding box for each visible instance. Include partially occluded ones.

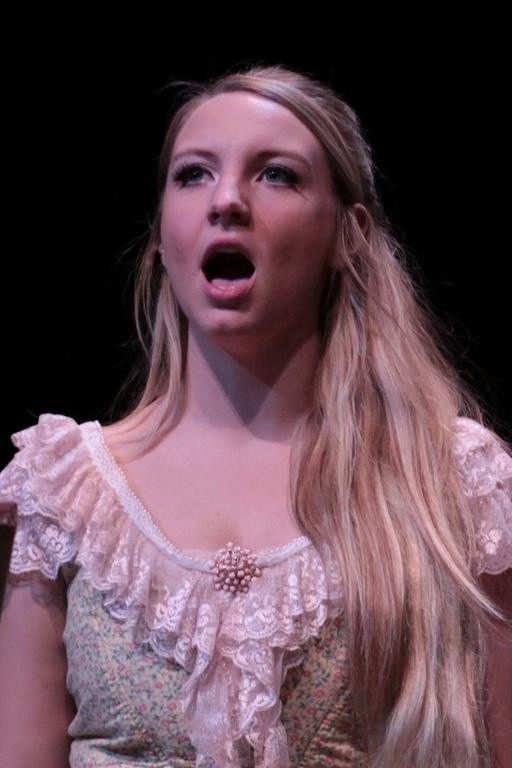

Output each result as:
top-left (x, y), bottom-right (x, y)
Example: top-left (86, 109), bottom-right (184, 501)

top-left (1, 62), bottom-right (512, 767)
